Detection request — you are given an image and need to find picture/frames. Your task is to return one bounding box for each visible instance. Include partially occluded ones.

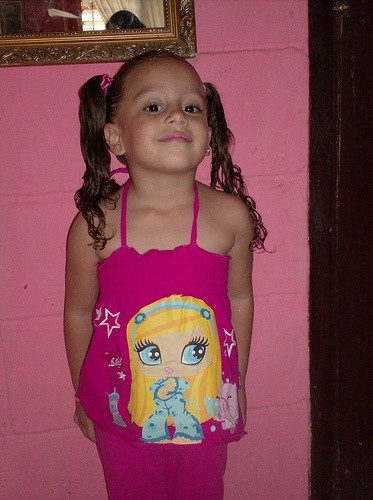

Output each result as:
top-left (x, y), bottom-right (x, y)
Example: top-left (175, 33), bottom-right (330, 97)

top-left (0, 0), bottom-right (198, 68)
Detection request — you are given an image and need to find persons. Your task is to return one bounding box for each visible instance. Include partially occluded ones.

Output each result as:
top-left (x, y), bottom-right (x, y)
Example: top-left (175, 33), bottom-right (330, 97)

top-left (63, 50), bottom-right (277, 500)
top-left (106, 10), bottom-right (147, 30)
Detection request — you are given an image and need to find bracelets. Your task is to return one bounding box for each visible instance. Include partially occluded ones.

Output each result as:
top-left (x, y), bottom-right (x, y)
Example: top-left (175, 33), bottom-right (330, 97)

top-left (74, 394), bottom-right (81, 404)
